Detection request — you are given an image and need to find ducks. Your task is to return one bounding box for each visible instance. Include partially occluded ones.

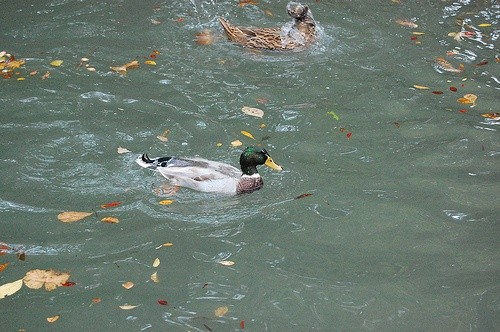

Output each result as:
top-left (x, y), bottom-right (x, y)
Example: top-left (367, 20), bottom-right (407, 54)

top-left (218, 5), bottom-right (316, 53)
top-left (136, 146), bottom-right (283, 195)
